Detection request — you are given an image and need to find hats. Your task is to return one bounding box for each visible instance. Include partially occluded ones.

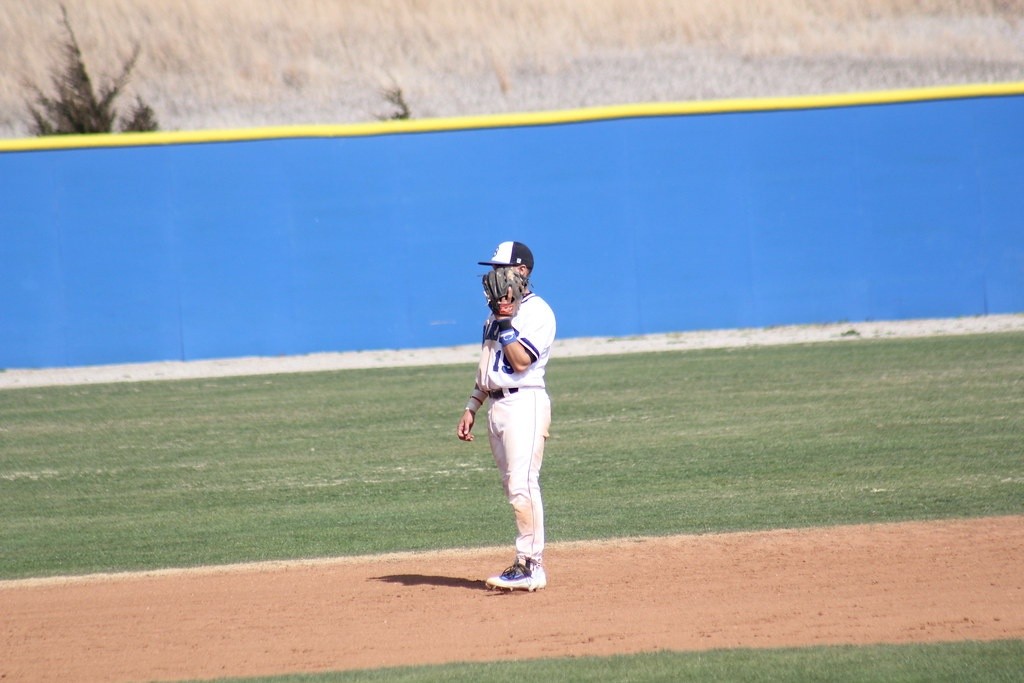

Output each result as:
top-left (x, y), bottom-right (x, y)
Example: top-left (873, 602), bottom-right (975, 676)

top-left (478, 241), bottom-right (534, 270)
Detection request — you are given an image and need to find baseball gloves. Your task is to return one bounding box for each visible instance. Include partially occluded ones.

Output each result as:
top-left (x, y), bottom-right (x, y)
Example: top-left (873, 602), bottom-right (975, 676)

top-left (481, 267), bottom-right (528, 319)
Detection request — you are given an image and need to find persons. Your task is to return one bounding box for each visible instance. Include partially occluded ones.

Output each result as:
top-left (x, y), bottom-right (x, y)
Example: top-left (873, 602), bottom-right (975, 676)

top-left (458, 241), bottom-right (557, 592)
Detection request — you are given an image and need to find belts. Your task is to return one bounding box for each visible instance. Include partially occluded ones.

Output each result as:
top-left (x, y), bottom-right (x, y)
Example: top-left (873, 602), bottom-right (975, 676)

top-left (487, 387), bottom-right (519, 398)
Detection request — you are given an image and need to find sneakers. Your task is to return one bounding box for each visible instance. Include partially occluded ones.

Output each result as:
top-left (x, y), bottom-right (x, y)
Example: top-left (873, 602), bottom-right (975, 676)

top-left (486, 560), bottom-right (548, 592)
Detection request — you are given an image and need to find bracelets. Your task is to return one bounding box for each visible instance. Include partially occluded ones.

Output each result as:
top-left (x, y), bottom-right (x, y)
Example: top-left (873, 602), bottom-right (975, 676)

top-left (498, 319), bottom-right (516, 346)
top-left (465, 389), bottom-right (488, 414)
